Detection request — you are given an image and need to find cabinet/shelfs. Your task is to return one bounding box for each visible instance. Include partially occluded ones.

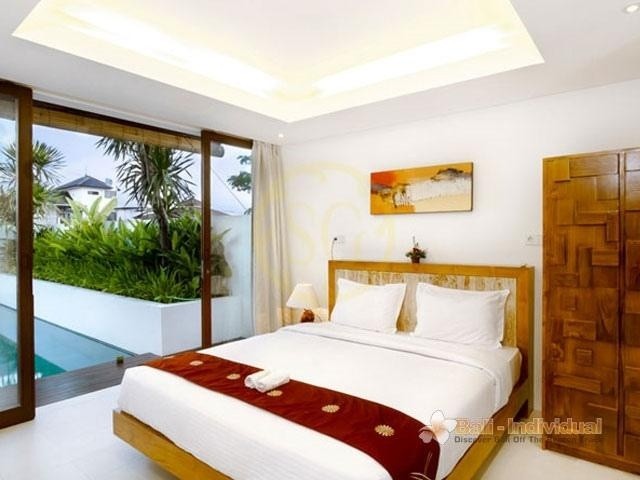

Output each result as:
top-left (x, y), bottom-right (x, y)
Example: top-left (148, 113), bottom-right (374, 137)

top-left (541, 147), bottom-right (640, 476)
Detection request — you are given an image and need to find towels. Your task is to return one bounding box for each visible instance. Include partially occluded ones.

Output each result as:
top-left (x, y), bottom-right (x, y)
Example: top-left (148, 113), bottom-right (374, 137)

top-left (256, 371), bottom-right (290, 393)
top-left (244, 368), bottom-right (272, 389)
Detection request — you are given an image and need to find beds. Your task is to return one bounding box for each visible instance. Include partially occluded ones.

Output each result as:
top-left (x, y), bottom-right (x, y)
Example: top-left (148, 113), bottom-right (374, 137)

top-left (113, 260), bottom-right (535, 480)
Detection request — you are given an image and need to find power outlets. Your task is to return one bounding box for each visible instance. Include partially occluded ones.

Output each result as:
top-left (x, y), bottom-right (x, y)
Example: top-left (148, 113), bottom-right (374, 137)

top-left (333, 236), bottom-right (342, 242)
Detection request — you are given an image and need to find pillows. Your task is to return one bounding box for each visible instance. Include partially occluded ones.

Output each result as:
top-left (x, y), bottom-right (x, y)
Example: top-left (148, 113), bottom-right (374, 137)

top-left (331, 279), bottom-right (407, 334)
top-left (410, 283), bottom-right (510, 350)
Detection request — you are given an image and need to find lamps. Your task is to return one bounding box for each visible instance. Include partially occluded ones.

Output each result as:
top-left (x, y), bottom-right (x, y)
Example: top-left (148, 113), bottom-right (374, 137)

top-left (286, 284), bottom-right (320, 322)
